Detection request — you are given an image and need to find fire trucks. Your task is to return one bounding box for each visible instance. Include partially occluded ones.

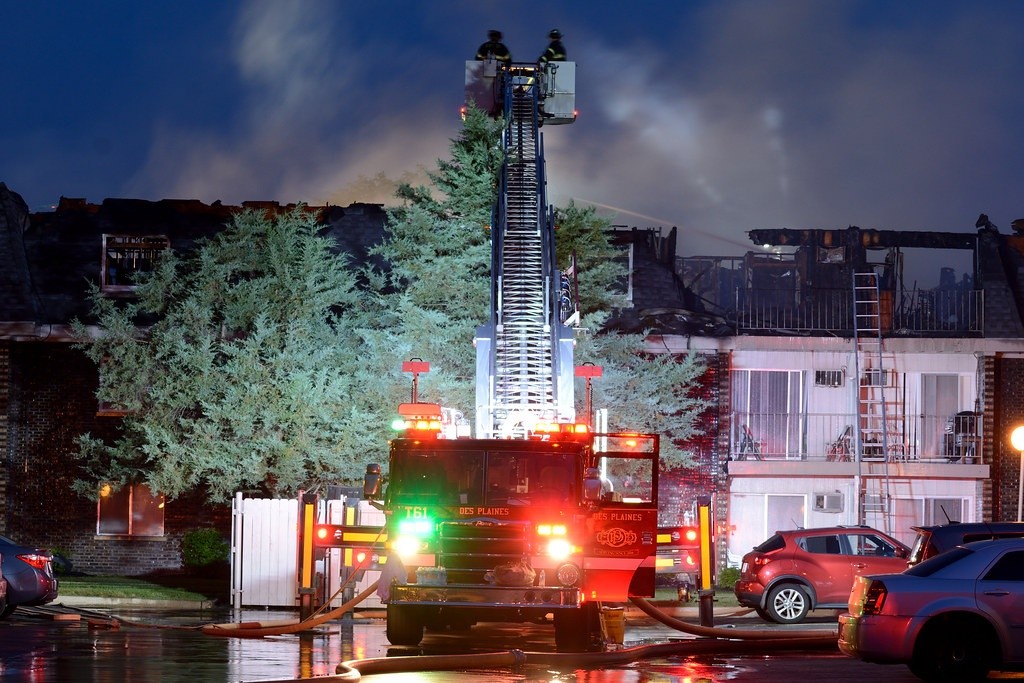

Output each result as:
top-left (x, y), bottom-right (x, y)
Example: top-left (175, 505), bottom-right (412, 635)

top-left (364, 60), bottom-right (660, 652)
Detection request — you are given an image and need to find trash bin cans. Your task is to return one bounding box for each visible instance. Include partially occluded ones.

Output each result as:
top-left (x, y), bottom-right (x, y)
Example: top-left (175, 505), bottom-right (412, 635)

top-left (599, 605), bottom-right (626, 644)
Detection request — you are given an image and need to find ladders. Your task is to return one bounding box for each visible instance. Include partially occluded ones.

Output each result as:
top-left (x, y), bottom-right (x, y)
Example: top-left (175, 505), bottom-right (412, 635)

top-left (852, 266), bottom-right (892, 555)
top-left (594, 407), bottom-right (608, 481)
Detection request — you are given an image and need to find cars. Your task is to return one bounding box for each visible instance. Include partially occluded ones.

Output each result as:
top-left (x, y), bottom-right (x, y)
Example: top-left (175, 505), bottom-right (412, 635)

top-left (838, 537), bottom-right (1024, 683)
top-left (734, 525), bottom-right (911, 623)
top-left (1, 534), bottom-right (58, 618)
top-left (907, 522), bottom-right (1024, 572)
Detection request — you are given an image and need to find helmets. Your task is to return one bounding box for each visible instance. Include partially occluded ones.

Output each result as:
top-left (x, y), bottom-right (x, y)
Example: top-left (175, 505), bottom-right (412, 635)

top-left (550, 29), bottom-right (560, 33)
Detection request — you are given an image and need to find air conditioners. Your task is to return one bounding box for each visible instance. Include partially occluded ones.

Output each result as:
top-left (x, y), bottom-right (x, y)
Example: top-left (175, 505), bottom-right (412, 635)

top-left (860, 494), bottom-right (892, 514)
top-left (861, 368), bottom-right (894, 388)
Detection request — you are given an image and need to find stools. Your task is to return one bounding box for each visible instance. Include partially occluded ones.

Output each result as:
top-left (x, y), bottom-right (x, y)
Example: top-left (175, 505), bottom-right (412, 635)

top-left (961, 436), bottom-right (981, 465)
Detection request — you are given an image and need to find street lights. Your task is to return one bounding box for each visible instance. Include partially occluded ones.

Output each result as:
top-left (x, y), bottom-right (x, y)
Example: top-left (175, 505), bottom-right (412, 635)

top-left (1011, 426), bottom-right (1024, 521)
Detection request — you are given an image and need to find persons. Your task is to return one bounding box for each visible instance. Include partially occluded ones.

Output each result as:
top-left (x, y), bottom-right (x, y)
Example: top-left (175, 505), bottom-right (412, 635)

top-left (475, 29), bottom-right (512, 65)
top-left (539, 29), bottom-right (566, 62)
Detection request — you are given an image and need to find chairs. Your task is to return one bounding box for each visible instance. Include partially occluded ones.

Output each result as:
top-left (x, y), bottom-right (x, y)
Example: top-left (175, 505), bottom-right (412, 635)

top-left (826, 425), bottom-right (854, 462)
top-left (738, 425), bottom-right (766, 461)
